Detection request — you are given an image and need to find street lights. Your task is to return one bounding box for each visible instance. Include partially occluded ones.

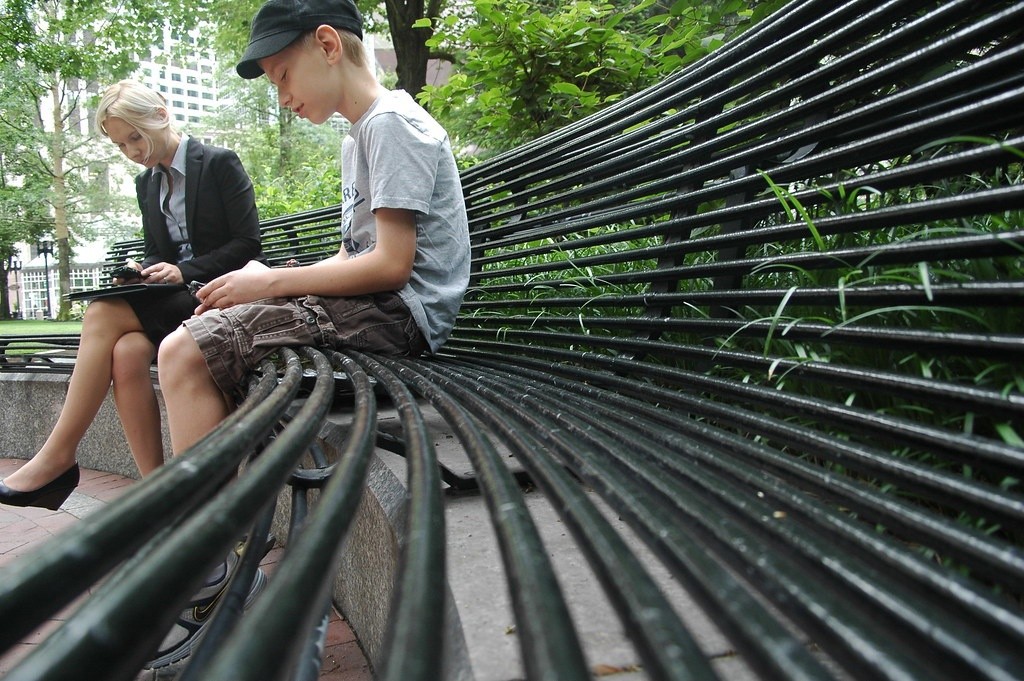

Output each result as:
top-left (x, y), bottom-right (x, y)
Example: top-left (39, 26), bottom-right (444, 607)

top-left (9, 260), bottom-right (23, 319)
top-left (37, 239), bottom-right (56, 320)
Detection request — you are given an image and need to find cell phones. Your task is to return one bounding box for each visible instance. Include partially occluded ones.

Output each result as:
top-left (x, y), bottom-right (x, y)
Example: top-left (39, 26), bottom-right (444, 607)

top-left (190, 280), bottom-right (205, 292)
top-left (110, 266), bottom-right (152, 281)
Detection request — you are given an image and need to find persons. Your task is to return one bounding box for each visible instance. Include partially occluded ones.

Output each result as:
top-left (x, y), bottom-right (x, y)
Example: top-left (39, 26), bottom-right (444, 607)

top-left (0, 80), bottom-right (262, 511)
top-left (145, 0), bottom-right (520, 670)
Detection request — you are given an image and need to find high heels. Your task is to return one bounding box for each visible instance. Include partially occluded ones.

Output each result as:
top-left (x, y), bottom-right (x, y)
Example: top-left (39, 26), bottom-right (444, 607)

top-left (0, 459), bottom-right (80, 511)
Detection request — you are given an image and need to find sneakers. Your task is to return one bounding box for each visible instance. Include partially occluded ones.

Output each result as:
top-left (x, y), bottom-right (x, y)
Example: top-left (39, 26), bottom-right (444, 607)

top-left (233, 527), bottom-right (276, 564)
top-left (141, 547), bottom-right (269, 670)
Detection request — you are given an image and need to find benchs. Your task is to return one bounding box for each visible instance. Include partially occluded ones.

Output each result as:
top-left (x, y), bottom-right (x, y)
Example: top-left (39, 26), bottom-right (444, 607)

top-left (0, 0), bottom-right (1024, 681)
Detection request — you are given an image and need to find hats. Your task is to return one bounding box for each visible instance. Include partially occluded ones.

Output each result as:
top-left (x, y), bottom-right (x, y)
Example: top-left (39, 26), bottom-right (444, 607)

top-left (235, 0), bottom-right (364, 79)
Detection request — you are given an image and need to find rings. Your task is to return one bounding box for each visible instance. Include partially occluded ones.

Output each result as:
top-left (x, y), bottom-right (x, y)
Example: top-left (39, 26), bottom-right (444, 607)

top-left (164, 277), bottom-right (167, 283)
